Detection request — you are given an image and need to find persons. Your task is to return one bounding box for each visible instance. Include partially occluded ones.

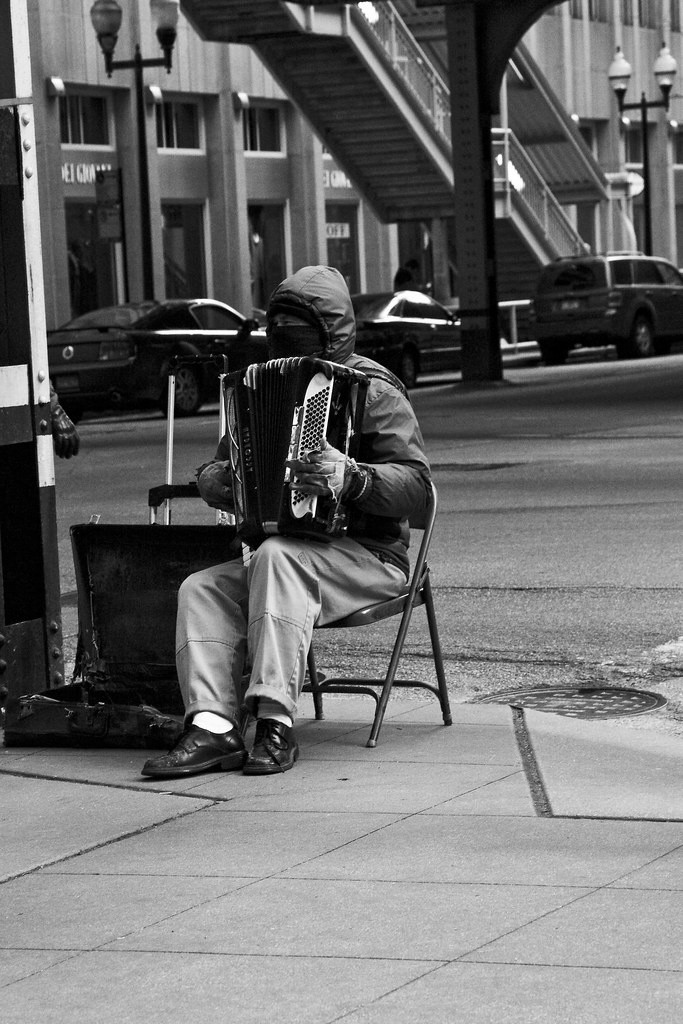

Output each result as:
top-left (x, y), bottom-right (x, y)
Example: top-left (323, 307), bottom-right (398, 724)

top-left (139, 263), bottom-right (432, 777)
top-left (51, 382), bottom-right (81, 460)
top-left (392, 258), bottom-right (432, 295)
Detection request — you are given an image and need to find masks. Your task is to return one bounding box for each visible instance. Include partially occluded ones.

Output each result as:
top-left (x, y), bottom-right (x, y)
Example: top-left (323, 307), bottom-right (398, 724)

top-left (269, 325), bottom-right (326, 358)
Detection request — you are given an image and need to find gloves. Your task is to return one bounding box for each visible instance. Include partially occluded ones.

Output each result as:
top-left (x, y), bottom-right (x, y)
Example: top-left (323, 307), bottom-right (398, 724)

top-left (197, 458), bottom-right (235, 513)
top-left (52, 405), bottom-right (81, 459)
top-left (291, 436), bottom-right (354, 507)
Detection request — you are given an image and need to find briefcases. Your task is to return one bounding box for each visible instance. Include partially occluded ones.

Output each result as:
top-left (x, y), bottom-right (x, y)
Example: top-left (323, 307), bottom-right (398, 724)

top-left (5, 514), bottom-right (243, 751)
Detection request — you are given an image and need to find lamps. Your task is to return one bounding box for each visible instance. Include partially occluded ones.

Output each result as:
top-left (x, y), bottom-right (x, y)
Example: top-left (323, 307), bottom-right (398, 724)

top-left (620, 116), bottom-right (632, 132)
top-left (48, 77), bottom-right (67, 98)
top-left (145, 85), bottom-right (165, 106)
top-left (233, 92), bottom-right (250, 110)
top-left (571, 114), bottom-right (580, 125)
top-left (668, 119), bottom-right (680, 134)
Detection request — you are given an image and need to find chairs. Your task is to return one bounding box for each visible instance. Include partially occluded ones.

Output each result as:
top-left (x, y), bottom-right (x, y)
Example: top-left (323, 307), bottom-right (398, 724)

top-left (296, 480), bottom-right (455, 752)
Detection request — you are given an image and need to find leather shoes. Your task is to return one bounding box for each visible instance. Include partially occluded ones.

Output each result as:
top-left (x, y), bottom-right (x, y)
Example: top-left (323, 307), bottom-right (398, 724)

top-left (141, 724), bottom-right (250, 775)
top-left (242, 719), bottom-right (300, 774)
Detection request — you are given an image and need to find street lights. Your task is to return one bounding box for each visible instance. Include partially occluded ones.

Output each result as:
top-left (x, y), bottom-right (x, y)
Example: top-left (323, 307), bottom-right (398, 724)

top-left (90, 0), bottom-right (181, 301)
top-left (605, 42), bottom-right (678, 261)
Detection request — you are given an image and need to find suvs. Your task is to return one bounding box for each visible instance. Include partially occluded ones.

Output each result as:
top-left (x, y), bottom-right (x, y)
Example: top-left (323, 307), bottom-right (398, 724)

top-left (536, 252), bottom-right (683, 363)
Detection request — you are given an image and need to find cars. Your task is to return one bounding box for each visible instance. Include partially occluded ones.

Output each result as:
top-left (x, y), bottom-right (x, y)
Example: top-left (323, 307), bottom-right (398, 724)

top-left (352, 287), bottom-right (464, 390)
top-left (52, 295), bottom-right (267, 421)
